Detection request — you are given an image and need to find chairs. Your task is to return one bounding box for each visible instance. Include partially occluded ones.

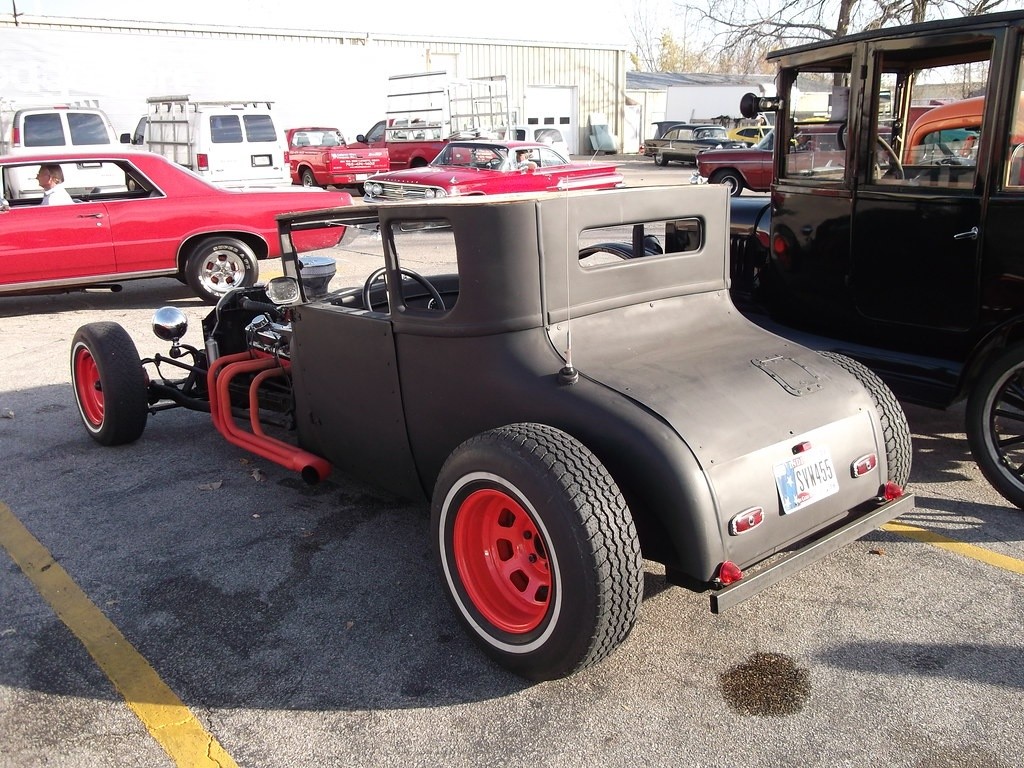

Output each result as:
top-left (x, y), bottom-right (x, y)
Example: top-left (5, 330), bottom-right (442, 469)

top-left (297, 133), bottom-right (310, 146)
top-left (72, 198), bottom-right (82, 203)
top-left (322, 134), bottom-right (335, 146)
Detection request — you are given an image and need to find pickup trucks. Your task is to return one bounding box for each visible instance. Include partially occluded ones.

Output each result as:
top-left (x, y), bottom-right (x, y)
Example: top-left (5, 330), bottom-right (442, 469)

top-left (347, 117), bottom-right (498, 172)
top-left (284, 127), bottom-right (391, 197)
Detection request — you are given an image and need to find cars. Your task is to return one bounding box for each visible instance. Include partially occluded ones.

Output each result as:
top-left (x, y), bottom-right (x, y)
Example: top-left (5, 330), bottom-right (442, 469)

top-left (0, 150), bottom-right (363, 307)
top-left (641, 90), bottom-right (1024, 197)
top-left (66, 181), bottom-right (916, 681)
top-left (664, 9), bottom-right (1023, 514)
top-left (362, 139), bottom-right (628, 204)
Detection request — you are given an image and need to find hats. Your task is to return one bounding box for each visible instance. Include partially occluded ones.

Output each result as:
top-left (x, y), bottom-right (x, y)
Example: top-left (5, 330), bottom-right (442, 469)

top-left (516, 150), bottom-right (528, 153)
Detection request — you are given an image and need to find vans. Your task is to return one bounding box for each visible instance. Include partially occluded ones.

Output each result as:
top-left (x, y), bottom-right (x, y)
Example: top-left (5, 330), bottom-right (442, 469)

top-left (121, 93), bottom-right (293, 188)
top-left (0, 98), bottom-right (129, 201)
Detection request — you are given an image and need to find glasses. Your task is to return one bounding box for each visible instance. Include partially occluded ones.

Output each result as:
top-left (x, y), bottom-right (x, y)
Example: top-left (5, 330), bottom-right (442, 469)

top-left (37, 174), bottom-right (48, 177)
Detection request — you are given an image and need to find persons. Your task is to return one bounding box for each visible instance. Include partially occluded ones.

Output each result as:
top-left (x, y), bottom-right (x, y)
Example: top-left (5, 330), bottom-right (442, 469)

top-left (516, 149), bottom-right (538, 169)
top-left (35, 164), bottom-right (75, 205)
top-left (789, 135), bottom-right (812, 152)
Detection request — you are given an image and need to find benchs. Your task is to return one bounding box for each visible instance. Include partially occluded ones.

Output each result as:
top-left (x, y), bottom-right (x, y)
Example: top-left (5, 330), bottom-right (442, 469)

top-left (149, 189), bottom-right (161, 198)
